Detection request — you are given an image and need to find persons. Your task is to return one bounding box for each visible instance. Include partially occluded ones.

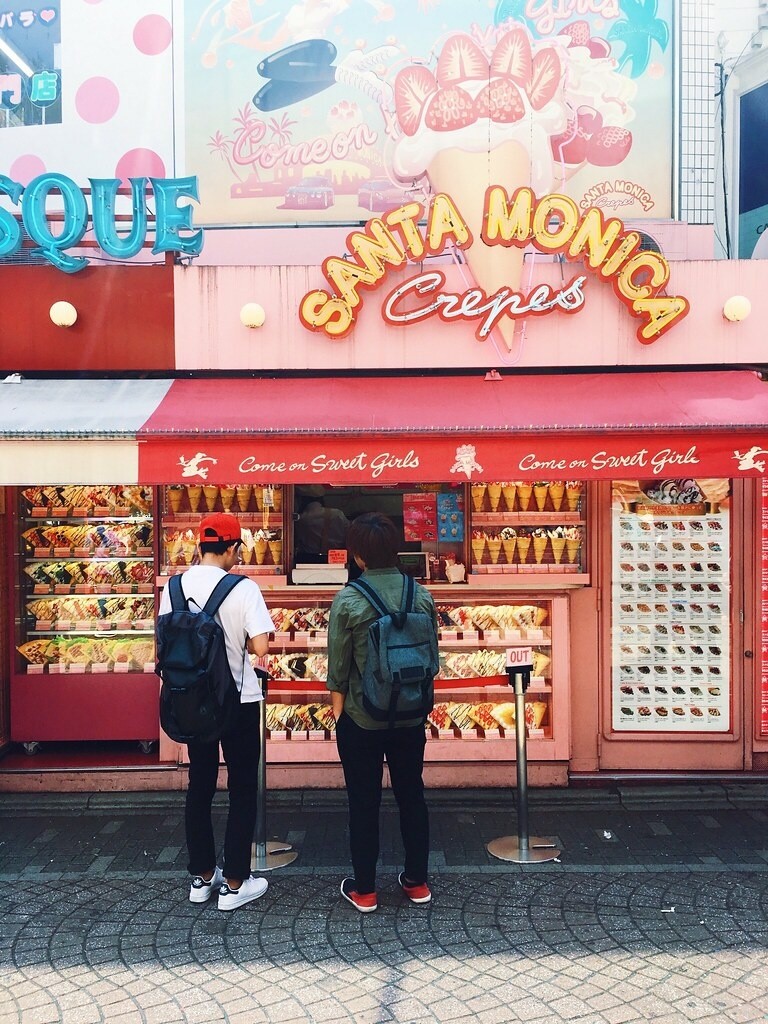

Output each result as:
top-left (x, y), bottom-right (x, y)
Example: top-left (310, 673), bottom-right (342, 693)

top-left (326, 512), bottom-right (439, 913)
top-left (294, 485), bottom-right (365, 580)
top-left (153, 512), bottom-right (276, 912)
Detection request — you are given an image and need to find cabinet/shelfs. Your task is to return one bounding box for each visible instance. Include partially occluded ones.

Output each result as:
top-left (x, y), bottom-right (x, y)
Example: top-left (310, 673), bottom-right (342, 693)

top-left (180, 593), bottom-right (573, 763)
top-left (6, 485), bottom-right (159, 756)
top-left (467, 480), bottom-right (590, 585)
top-left (155, 484), bottom-right (287, 587)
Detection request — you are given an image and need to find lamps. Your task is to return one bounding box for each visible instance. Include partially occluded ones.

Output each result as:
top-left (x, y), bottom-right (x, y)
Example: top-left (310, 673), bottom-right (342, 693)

top-left (49, 300), bottom-right (78, 328)
top-left (721, 295), bottom-right (752, 322)
top-left (240, 304), bottom-right (266, 327)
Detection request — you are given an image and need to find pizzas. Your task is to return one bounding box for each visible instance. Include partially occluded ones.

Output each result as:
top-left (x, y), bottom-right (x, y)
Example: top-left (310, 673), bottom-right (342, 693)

top-left (16, 486), bottom-right (153, 665)
top-left (262, 605), bottom-right (552, 732)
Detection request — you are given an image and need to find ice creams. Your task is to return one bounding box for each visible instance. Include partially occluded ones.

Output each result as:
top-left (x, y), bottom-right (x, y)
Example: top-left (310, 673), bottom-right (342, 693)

top-left (385, 19), bottom-right (580, 364)
top-left (164, 484), bottom-right (281, 565)
top-left (470, 480), bottom-right (583, 565)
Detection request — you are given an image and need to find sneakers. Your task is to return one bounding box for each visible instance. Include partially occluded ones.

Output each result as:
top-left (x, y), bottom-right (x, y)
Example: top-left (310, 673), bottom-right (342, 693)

top-left (340, 877), bottom-right (377, 912)
top-left (218, 875), bottom-right (268, 910)
top-left (398, 871), bottom-right (431, 902)
top-left (189, 865), bottom-right (225, 902)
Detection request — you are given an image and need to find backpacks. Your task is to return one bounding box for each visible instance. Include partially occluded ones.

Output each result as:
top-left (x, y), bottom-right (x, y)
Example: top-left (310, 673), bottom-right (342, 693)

top-left (345, 574), bottom-right (440, 722)
top-left (154, 573), bottom-right (248, 745)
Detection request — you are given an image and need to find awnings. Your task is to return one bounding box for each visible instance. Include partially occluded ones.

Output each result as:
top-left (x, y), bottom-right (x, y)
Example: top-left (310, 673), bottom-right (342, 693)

top-left (0, 369), bottom-right (768, 486)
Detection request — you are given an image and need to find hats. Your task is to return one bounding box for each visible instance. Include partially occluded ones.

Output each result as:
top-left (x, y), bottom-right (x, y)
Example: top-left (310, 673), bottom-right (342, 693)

top-left (199, 512), bottom-right (247, 547)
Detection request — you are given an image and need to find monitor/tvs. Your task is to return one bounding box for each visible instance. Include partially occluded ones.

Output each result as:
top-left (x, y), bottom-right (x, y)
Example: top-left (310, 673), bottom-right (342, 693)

top-left (396, 552), bottom-right (431, 580)
